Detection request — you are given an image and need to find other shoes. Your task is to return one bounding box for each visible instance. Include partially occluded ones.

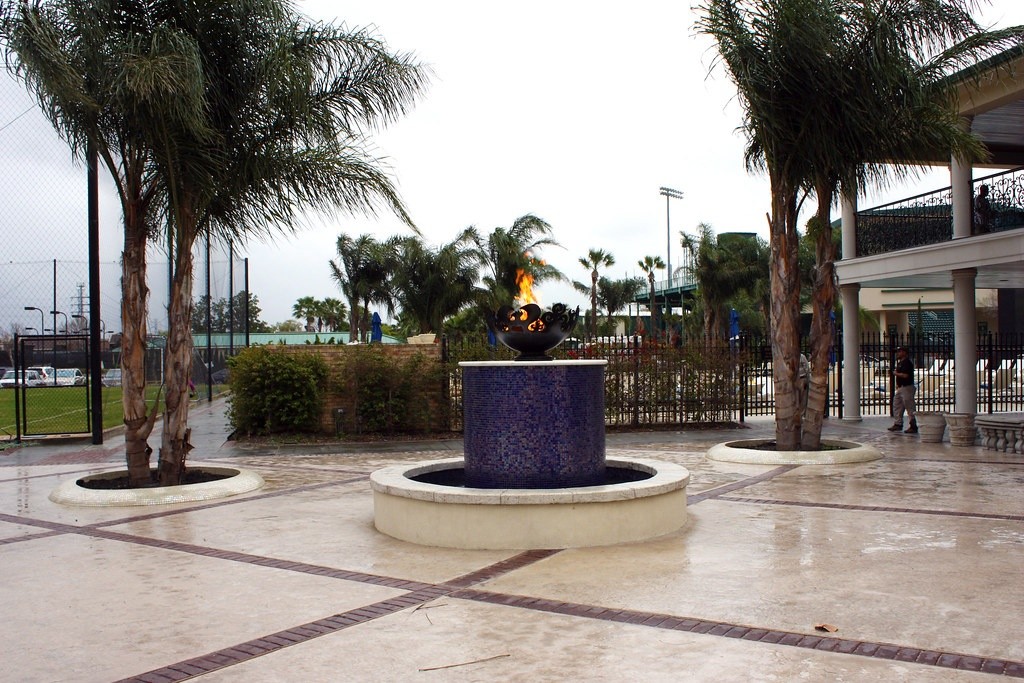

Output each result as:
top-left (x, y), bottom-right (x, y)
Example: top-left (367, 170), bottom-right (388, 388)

top-left (904, 427), bottom-right (919, 433)
top-left (887, 424), bottom-right (903, 430)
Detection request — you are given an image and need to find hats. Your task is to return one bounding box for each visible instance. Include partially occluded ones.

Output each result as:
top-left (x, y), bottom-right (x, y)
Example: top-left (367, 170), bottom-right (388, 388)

top-left (894, 346), bottom-right (907, 352)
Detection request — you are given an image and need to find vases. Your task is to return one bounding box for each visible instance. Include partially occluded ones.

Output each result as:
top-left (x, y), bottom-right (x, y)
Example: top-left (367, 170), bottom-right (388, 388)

top-left (912, 411), bottom-right (950, 443)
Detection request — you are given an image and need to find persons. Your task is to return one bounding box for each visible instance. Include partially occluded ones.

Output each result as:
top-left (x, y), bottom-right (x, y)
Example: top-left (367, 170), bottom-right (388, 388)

top-left (799, 354), bottom-right (810, 419)
top-left (973, 185), bottom-right (991, 234)
top-left (189, 381), bottom-right (195, 395)
top-left (888, 346), bottom-right (918, 434)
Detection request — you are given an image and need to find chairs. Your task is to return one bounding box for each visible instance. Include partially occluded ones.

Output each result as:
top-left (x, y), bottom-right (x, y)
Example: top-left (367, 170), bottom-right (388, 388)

top-left (860, 358), bottom-right (1024, 401)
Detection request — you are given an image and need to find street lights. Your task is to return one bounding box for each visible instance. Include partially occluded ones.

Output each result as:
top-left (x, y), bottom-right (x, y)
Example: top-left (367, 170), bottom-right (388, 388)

top-left (49, 310), bottom-right (69, 363)
top-left (71, 315), bottom-right (91, 433)
top-left (25, 327), bottom-right (40, 349)
top-left (659, 185), bottom-right (687, 288)
top-left (24, 306), bottom-right (45, 368)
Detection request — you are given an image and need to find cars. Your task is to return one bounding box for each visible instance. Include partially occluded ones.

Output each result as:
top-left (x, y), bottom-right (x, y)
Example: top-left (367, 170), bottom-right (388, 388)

top-left (0, 369), bottom-right (47, 387)
top-left (102, 368), bottom-right (122, 386)
top-left (28, 365), bottom-right (56, 382)
top-left (44, 368), bottom-right (85, 386)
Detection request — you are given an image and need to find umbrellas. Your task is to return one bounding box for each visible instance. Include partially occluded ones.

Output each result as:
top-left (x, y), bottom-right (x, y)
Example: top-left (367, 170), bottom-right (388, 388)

top-left (828, 310), bottom-right (836, 366)
top-left (729, 308), bottom-right (740, 356)
top-left (370, 312), bottom-right (382, 343)
top-left (488, 329), bottom-right (497, 345)
